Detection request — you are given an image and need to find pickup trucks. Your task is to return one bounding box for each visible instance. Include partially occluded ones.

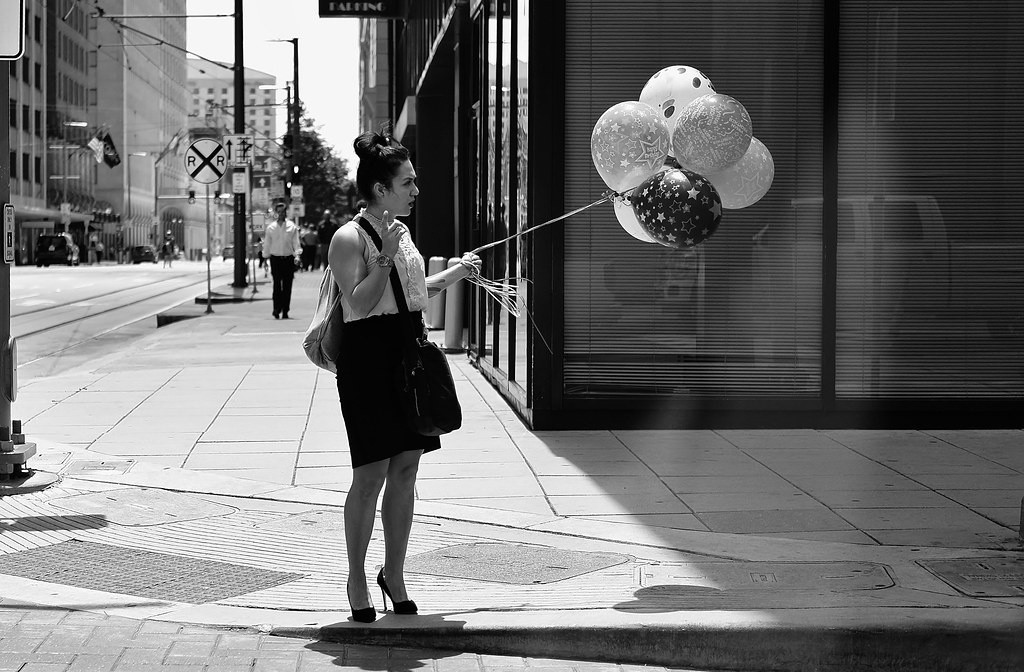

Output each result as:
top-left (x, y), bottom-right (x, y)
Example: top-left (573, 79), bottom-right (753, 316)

top-left (795, 194), bottom-right (1024, 353)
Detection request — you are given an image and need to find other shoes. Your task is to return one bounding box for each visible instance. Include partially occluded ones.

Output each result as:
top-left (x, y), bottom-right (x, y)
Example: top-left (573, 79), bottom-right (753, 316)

top-left (273, 310), bottom-right (279, 319)
top-left (283, 312), bottom-right (288, 318)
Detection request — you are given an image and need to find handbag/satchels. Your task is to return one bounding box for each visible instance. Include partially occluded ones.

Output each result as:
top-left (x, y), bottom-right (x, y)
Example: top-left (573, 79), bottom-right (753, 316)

top-left (402, 336), bottom-right (461, 436)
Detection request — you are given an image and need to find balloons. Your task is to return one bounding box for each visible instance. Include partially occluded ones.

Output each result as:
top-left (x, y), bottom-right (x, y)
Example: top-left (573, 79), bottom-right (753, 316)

top-left (591, 65), bottom-right (774, 249)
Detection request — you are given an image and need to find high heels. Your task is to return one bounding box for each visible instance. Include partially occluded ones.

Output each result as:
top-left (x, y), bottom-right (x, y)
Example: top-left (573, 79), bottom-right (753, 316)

top-left (377, 566), bottom-right (417, 613)
top-left (347, 574), bottom-right (376, 623)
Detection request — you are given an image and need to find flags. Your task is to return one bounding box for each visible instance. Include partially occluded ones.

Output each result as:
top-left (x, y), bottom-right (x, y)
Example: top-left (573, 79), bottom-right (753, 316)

top-left (99, 133), bottom-right (121, 169)
top-left (87, 137), bottom-right (104, 164)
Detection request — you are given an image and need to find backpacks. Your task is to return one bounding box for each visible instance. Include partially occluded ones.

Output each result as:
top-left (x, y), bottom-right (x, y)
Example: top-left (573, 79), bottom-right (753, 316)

top-left (302, 265), bottom-right (343, 374)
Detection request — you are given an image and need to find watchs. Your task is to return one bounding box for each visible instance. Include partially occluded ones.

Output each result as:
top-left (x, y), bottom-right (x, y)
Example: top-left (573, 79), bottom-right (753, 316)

top-left (376, 254), bottom-right (394, 268)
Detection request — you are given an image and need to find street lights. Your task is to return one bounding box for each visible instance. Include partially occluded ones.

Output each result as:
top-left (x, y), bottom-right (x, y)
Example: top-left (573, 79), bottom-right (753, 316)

top-left (266, 38), bottom-right (299, 225)
top-left (259, 84), bottom-right (291, 211)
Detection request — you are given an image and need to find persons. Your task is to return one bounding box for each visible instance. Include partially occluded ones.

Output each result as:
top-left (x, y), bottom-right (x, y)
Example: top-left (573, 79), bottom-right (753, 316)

top-left (257, 236), bottom-right (263, 268)
top-left (299, 201), bottom-right (368, 272)
top-left (262, 203), bottom-right (303, 319)
top-left (89, 241), bottom-right (96, 265)
top-left (96, 241), bottom-right (103, 264)
top-left (329, 134), bottom-right (482, 622)
top-left (163, 237), bottom-right (173, 268)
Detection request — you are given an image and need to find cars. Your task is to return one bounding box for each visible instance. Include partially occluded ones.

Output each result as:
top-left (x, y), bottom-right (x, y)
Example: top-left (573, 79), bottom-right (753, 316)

top-left (133, 245), bottom-right (158, 264)
top-left (33, 232), bottom-right (79, 268)
top-left (222, 246), bottom-right (235, 261)
